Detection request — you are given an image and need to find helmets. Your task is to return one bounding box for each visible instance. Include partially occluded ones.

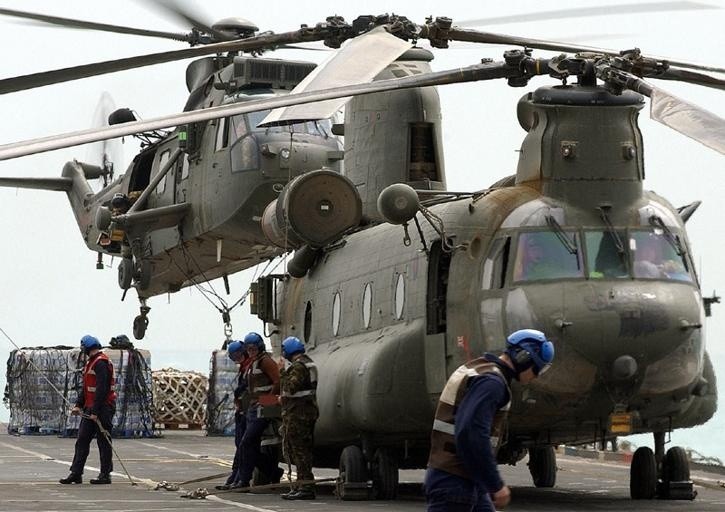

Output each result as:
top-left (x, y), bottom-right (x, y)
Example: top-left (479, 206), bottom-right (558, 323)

top-left (506, 328), bottom-right (556, 375)
top-left (81, 335), bottom-right (101, 354)
top-left (244, 332), bottom-right (265, 351)
top-left (280, 335), bottom-right (305, 359)
top-left (229, 339), bottom-right (244, 360)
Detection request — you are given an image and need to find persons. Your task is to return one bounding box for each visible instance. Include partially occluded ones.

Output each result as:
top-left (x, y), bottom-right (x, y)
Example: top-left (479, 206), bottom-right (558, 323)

top-left (277, 335), bottom-right (320, 499)
top-left (522, 238), bottom-right (556, 279)
top-left (232, 332), bottom-right (280, 489)
top-left (215, 340), bottom-right (253, 490)
top-left (423, 329), bottom-right (555, 512)
top-left (110, 188), bottom-right (143, 219)
top-left (59, 335), bottom-right (117, 485)
top-left (636, 236), bottom-right (671, 279)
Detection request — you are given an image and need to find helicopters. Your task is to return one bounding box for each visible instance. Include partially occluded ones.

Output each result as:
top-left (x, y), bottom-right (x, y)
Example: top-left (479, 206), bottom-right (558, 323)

top-left (0, 1), bottom-right (720, 344)
top-left (1, 44), bottom-right (721, 501)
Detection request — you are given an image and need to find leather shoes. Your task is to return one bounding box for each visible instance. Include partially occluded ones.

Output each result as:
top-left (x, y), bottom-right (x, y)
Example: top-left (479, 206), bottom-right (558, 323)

top-left (90, 475), bottom-right (113, 485)
top-left (280, 489), bottom-right (316, 501)
top-left (60, 472), bottom-right (82, 484)
top-left (217, 476), bottom-right (250, 493)
